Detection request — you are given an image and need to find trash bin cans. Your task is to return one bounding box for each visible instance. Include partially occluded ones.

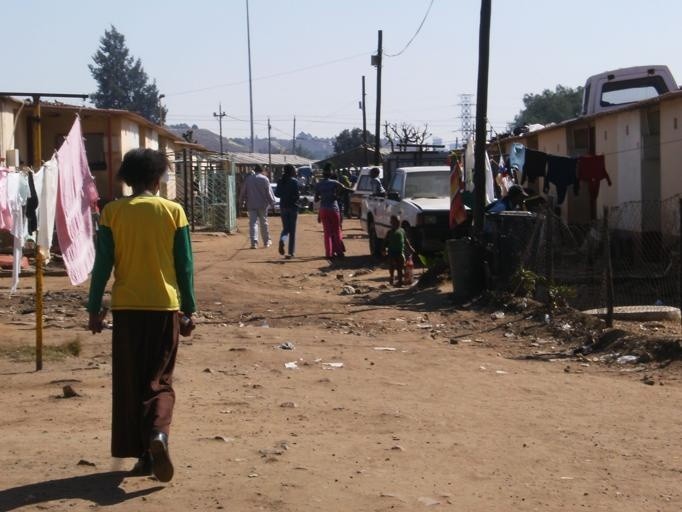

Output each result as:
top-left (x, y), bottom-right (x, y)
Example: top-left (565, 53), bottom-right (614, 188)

top-left (446, 238), bottom-right (482, 298)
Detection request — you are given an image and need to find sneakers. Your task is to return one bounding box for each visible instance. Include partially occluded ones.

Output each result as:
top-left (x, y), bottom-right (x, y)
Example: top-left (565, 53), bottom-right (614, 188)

top-left (251, 243), bottom-right (257, 249)
top-left (264, 240), bottom-right (272, 247)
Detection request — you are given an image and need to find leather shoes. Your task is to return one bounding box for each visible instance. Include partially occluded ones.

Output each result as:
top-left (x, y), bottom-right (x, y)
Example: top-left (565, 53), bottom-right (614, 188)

top-left (151, 432), bottom-right (173, 483)
top-left (134, 452), bottom-right (153, 476)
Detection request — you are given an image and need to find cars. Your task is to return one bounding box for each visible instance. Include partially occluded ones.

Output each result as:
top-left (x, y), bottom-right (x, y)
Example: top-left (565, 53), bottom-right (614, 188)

top-left (268, 183), bottom-right (286, 215)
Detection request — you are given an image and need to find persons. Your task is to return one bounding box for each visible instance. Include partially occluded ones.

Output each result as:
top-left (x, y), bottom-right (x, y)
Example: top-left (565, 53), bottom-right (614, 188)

top-left (298, 163), bottom-right (358, 258)
top-left (382, 215), bottom-right (415, 285)
top-left (239, 164), bottom-right (275, 248)
top-left (370, 168), bottom-right (385, 197)
top-left (275, 164), bottom-right (300, 257)
top-left (87, 149), bottom-right (197, 483)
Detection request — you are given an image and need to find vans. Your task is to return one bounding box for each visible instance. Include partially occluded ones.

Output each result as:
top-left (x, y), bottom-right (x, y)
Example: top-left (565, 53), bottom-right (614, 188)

top-left (578, 64), bottom-right (679, 118)
top-left (345, 166), bottom-right (384, 218)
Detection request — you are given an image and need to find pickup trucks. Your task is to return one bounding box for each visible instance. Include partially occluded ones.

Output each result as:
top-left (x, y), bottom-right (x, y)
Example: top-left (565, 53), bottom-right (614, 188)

top-left (359, 165), bottom-right (475, 258)
